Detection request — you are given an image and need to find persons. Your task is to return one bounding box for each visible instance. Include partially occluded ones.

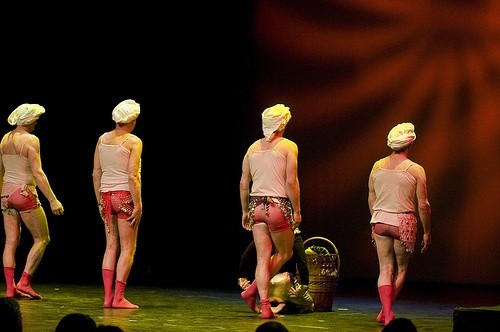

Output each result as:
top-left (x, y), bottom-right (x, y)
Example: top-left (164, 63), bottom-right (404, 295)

top-left (0, 296), bottom-right (418, 332)
top-left (0, 102), bottom-right (65, 298)
top-left (92, 99), bottom-right (143, 309)
top-left (241, 104), bottom-right (314, 318)
top-left (368, 122), bottom-right (432, 325)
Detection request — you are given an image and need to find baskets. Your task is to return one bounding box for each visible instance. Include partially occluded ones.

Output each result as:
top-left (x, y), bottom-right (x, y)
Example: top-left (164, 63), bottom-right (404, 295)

top-left (295, 237), bottom-right (339, 311)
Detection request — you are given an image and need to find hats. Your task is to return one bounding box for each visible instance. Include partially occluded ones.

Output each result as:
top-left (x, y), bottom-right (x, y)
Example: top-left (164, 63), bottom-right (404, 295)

top-left (112, 99), bottom-right (141, 124)
top-left (8, 103), bottom-right (46, 126)
top-left (386, 122), bottom-right (417, 150)
top-left (261, 104), bottom-right (291, 143)
